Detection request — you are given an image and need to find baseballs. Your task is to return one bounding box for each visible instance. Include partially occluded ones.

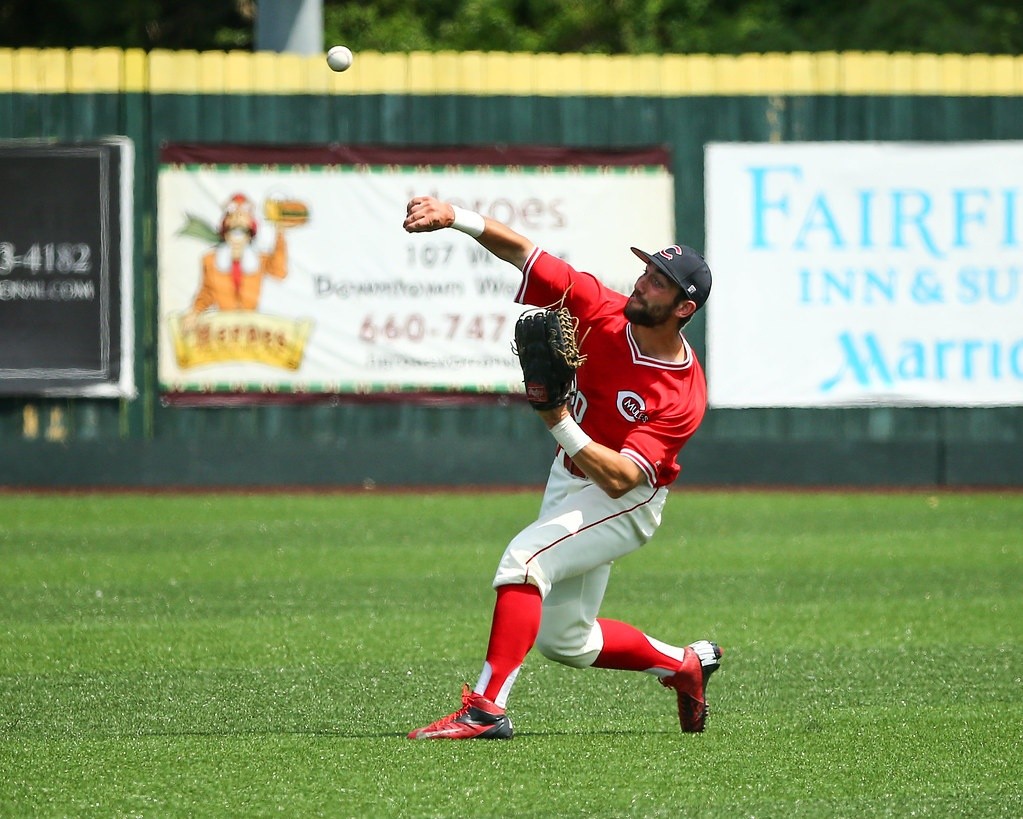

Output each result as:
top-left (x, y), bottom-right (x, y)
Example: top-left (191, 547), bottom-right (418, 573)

top-left (326, 46), bottom-right (353, 72)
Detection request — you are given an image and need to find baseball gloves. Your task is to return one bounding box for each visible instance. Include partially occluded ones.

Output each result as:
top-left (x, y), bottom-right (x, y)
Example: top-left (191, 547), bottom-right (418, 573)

top-left (508, 280), bottom-right (592, 412)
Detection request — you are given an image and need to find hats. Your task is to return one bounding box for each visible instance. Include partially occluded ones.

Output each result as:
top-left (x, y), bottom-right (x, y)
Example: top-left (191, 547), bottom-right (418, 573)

top-left (630, 245), bottom-right (713, 313)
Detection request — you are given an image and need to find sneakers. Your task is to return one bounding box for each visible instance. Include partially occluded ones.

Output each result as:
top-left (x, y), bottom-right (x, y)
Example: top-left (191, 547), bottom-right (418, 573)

top-left (657, 640), bottom-right (723, 733)
top-left (406, 683), bottom-right (514, 740)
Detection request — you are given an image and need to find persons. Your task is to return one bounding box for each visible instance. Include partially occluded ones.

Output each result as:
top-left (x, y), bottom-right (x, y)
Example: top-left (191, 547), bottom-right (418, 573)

top-left (403, 196), bottom-right (722, 740)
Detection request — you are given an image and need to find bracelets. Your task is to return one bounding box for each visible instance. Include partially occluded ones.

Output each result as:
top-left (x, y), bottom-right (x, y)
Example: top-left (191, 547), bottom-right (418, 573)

top-left (450, 206), bottom-right (484, 239)
top-left (550, 413), bottom-right (591, 458)
